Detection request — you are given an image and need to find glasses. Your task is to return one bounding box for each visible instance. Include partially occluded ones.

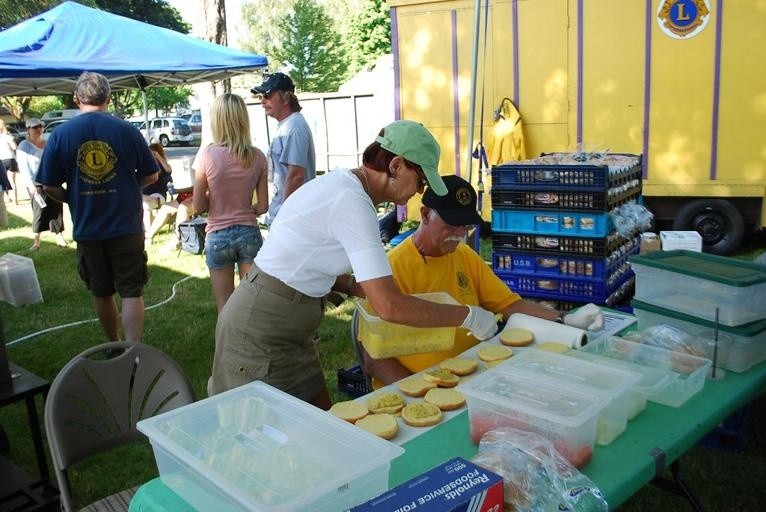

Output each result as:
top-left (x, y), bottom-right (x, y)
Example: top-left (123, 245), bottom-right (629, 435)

top-left (413, 168), bottom-right (428, 186)
top-left (256, 93), bottom-right (272, 99)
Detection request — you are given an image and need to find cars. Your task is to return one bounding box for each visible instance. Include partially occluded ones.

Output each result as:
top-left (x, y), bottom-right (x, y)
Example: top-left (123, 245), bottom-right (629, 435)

top-left (128, 121), bottom-right (145, 129)
top-left (4, 118), bottom-right (73, 146)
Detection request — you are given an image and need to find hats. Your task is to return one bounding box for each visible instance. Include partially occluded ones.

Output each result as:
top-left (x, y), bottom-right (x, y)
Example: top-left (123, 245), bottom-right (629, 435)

top-left (374, 118), bottom-right (449, 197)
top-left (422, 173), bottom-right (486, 226)
top-left (250, 73), bottom-right (294, 96)
top-left (25, 117), bottom-right (45, 128)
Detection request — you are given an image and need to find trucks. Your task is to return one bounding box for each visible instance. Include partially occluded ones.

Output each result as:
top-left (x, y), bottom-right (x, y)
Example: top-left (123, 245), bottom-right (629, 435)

top-left (387, 0), bottom-right (766, 261)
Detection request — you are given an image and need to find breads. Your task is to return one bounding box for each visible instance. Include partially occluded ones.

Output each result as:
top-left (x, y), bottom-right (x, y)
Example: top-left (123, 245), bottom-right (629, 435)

top-left (329, 327), bottom-right (569, 440)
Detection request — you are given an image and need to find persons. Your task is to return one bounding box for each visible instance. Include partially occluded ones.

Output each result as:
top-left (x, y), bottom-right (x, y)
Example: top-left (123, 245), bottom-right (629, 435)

top-left (142, 143), bottom-right (172, 230)
top-left (250, 73), bottom-right (316, 226)
top-left (206, 121), bottom-right (500, 411)
top-left (35, 71), bottom-right (159, 342)
top-left (0, 119), bottom-right (20, 206)
top-left (0, 159), bottom-right (12, 228)
top-left (144, 194), bottom-right (193, 256)
top-left (186, 92), bottom-right (269, 313)
top-left (15, 118), bottom-right (67, 252)
top-left (357, 177), bottom-right (606, 387)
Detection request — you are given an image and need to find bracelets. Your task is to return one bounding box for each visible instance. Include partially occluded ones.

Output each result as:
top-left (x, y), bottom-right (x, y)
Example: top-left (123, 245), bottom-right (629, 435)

top-left (556, 312), bottom-right (565, 323)
top-left (346, 274), bottom-right (357, 297)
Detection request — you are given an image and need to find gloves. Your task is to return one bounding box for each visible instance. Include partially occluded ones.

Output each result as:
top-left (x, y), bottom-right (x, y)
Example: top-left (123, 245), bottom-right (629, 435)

top-left (460, 302), bottom-right (500, 342)
top-left (564, 303), bottom-right (605, 332)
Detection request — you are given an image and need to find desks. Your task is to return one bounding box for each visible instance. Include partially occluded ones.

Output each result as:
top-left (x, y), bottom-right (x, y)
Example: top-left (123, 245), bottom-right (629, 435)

top-left (0, 351), bottom-right (62, 512)
top-left (129, 304), bottom-right (765, 512)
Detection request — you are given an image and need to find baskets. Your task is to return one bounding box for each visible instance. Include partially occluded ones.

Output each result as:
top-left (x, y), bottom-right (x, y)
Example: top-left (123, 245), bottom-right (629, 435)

top-left (490, 150), bottom-right (641, 305)
top-left (337, 364), bottom-right (368, 398)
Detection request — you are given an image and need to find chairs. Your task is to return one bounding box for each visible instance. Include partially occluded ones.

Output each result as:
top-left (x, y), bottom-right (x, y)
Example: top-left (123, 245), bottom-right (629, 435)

top-left (43, 334), bottom-right (199, 512)
top-left (350, 306), bottom-right (374, 393)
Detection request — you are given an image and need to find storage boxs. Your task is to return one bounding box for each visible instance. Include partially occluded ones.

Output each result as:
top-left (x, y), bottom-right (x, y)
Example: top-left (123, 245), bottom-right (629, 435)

top-left (623, 296), bottom-right (765, 375)
top-left (0, 251), bottom-right (44, 310)
top-left (454, 333), bottom-right (710, 474)
top-left (353, 291), bottom-right (462, 359)
top-left (135, 377), bottom-right (405, 512)
top-left (178, 221), bottom-right (212, 255)
top-left (617, 247), bottom-right (765, 327)
top-left (638, 231), bottom-right (661, 254)
top-left (342, 456), bottom-right (504, 512)
top-left (659, 228), bottom-right (704, 253)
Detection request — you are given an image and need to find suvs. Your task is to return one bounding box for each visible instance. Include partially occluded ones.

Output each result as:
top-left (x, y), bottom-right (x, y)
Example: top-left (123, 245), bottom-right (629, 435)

top-left (137, 117), bottom-right (193, 147)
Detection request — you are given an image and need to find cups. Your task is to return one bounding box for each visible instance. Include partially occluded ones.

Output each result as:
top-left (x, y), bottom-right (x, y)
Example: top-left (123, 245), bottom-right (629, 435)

top-left (698, 331), bottom-right (734, 382)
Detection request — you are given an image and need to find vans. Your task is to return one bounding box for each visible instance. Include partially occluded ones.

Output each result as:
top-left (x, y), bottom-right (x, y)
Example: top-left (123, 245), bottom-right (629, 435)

top-left (180, 114), bottom-right (202, 133)
top-left (37, 108), bottom-right (82, 128)
